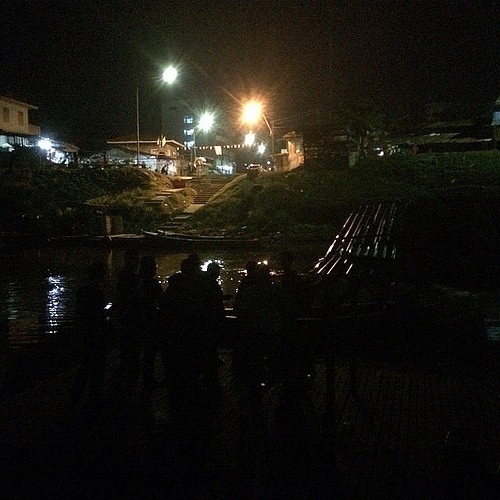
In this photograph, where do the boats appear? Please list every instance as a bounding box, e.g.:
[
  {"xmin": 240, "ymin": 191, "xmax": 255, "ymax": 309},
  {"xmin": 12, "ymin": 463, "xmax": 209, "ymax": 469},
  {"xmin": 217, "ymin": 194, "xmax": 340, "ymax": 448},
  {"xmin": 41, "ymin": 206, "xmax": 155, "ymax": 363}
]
[{"xmin": 155, "ymin": 229, "xmax": 260, "ymax": 251}]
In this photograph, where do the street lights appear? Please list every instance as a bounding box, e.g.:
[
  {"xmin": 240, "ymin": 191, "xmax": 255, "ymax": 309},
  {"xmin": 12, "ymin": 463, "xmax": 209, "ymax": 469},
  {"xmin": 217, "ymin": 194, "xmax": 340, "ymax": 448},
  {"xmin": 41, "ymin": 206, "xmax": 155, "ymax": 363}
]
[
  {"xmin": 246, "ymin": 100, "xmax": 276, "ymax": 172},
  {"xmin": 189, "ymin": 111, "xmax": 212, "ymax": 175},
  {"xmin": 136, "ymin": 66, "xmax": 178, "ymax": 169}
]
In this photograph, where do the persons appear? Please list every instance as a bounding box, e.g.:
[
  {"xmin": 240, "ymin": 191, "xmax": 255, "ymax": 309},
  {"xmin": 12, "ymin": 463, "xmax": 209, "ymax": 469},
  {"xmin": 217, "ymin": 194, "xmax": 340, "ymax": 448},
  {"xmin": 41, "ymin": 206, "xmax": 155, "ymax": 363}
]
[
  {"xmin": 67, "ymin": 261, "xmax": 109, "ymax": 417},
  {"xmin": 116, "ymin": 248, "xmax": 140, "ymax": 375},
  {"xmin": 159, "ymin": 253, "xmax": 227, "ymax": 400},
  {"xmin": 121, "ymin": 255, "xmax": 165, "ymax": 390},
  {"xmin": 237, "ymin": 250, "xmax": 365, "ymax": 409},
  {"xmin": 140, "ymin": 161, "xmax": 168, "ymax": 176}
]
